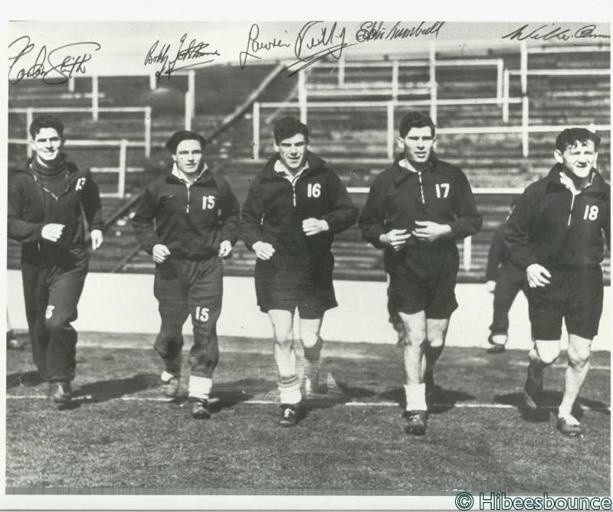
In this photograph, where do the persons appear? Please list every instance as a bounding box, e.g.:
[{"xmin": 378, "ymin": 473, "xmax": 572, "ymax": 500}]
[
  {"xmin": 357, "ymin": 111, "xmax": 483, "ymax": 434},
  {"xmin": 487, "ymin": 200, "xmax": 529, "ymax": 345},
  {"xmin": 503, "ymin": 128, "xmax": 610, "ymax": 437},
  {"xmin": 132, "ymin": 131, "xmax": 240, "ymax": 418},
  {"xmin": 8, "ymin": 120, "xmax": 104, "ymax": 402},
  {"xmin": 238, "ymin": 115, "xmax": 360, "ymax": 427}
]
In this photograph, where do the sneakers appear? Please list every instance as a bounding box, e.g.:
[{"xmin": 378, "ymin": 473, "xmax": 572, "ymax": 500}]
[
  {"xmin": 39, "ymin": 379, "xmax": 71, "ymax": 402},
  {"xmin": 488, "ymin": 331, "xmax": 507, "ymax": 347},
  {"xmin": 158, "ymin": 367, "xmax": 180, "ymax": 396},
  {"xmin": 300, "ymin": 365, "xmax": 319, "ymax": 394},
  {"xmin": 548, "ymin": 406, "xmax": 583, "ymax": 437},
  {"xmin": 7, "ymin": 337, "xmax": 24, "ymax": 350},
  {"xmin": 524, "ymin": 364, "xmax": 544, "ymax": 409},
  {"xmin": 278, "ymin": 401, "xmax": 303, "ymax": 425},
  {"xmin": 191, "ymin": 395, "xmax": 210, "ymax": 418},
  {"xmin": 403, "ymin": 409, "xmax": 427, "ymax": 435}
]
